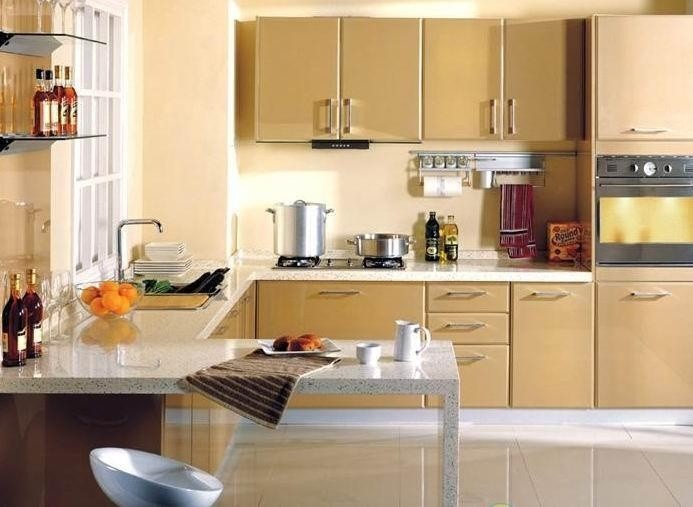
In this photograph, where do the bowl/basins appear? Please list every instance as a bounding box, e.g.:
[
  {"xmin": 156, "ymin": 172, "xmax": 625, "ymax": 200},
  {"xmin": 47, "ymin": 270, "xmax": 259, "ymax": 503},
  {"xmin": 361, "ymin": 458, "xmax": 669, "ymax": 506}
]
[
  {"xmin": 75, "ymin": 281, "xmax": 146, "ymax": 320},
  {"xmin": 80, "ymin": 320, "xmax": 142, "ymax": 349}
]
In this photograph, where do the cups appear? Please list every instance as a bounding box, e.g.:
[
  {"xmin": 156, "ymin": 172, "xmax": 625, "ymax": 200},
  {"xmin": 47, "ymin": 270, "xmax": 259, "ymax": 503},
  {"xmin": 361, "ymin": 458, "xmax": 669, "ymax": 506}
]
[
  {"xmin": 357, "ymin": 342, "xmax": 381, "ymax": 364},
  {"xmin": 392, "ymin": 320, "xmax": 431, "ymax": 362},
  {"xmin": 41, "ymin": 274, "xmax": 62, "ymax": 345},
  {"xmin": 37, "ymin": 277, "xmax": 51, "ymax": 349},
  {"xmin": 50, "ymin": 269, "xmax": 73, "ymax": 340}
]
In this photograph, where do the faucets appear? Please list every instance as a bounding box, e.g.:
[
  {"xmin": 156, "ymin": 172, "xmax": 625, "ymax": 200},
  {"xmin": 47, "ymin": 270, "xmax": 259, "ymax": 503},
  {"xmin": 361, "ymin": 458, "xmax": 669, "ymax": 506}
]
[{"xmin": 114, "ymin": 219, "xmax": 163, "ymax": 281}]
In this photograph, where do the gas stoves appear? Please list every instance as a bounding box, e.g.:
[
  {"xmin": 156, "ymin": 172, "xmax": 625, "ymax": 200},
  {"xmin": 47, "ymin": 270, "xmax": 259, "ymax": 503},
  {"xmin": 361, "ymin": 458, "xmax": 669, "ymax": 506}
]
[{"xmin": 271, "ymin": 255, "xmax": 407, "ymax": 271}]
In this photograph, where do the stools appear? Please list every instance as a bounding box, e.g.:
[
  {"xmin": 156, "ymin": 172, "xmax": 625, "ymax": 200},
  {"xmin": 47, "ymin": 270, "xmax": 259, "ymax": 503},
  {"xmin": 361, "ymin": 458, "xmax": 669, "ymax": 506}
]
[{"xmin": 90, "ymin": 447, "xmax": 223, "ymax": 506}]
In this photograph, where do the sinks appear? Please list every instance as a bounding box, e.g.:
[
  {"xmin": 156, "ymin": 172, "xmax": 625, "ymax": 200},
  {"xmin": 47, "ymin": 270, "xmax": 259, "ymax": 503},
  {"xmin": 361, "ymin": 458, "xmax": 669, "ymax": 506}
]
[{"xmin": 144, "ymin": 282, "xmax": 221, "ymax": 309}]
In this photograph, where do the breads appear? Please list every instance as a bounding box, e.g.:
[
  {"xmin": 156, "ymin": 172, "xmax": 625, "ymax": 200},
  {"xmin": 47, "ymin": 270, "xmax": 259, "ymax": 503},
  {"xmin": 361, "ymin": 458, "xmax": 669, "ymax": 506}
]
[
  {"xmin": 273, "ymin": 334, "xmax": 299, "ymax": 352},
  {"xmin": 301, "ymin": 334, "xmax": 321, "ymax": 348},
  {"xmin": 288, "ymin": 337, "xmax": 314, "ymax": 353}
]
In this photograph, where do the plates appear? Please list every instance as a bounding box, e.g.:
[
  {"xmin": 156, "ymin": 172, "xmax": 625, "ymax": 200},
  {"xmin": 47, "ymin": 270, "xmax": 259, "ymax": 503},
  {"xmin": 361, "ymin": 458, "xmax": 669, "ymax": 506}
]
[
  {"xmin": 144, "ymin": 242, "xmax": 186, "ymax": 261},
  {"xmin": 256, "ymin": 337, "xmax": 342, "ymax": 354},
  {"xmin": 134, "ymin": 254, "xmax": 193, "ymax": 278}
]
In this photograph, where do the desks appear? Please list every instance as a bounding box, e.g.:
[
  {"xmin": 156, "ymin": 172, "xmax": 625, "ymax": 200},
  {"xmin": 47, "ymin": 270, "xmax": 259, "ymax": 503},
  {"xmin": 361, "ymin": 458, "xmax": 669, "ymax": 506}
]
[{"xmin": 1, "ymin": 339, "xmax": 461, "ymax": 507}]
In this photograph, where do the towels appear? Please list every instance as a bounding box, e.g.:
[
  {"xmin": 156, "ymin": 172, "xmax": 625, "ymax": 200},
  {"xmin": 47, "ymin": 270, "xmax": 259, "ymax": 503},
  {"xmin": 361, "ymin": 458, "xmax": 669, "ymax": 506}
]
[
  {"xmin": 498, "ymin": 182, "xmax": 539, "ymax": 259},
  {"xmin": 183, "ymin": 345, "xmax": 342, "ymax": 431}
]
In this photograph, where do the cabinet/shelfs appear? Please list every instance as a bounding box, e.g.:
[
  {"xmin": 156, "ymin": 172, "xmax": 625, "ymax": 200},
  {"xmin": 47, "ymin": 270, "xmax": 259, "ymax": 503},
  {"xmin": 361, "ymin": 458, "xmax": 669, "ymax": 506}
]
[
  {"xmin": 0, "ymin": 132, "xmax": 107, "ymax": 153},
  {"xmin": 426, "ymin": 284, "xmax": 509, "ymax": 312},
  {"xmin": 512, "ymin": 284, "xmax": 596, "ymax": 408},
  {"xmin": 256, "ymin": 18, "xmax": 422, "ymax": 142},
  {"xmin": 591, "ymin": 14, "xmax": 693, "ymax": 142},
  {"xmin": 426, "ymin": 344, "xmax": 510, "ymax": 406},
  {"xmin": 594, "ymin": 279, "xmax": 692, "ymax": 408},
  {"xmin": 258, "ymin": 281, "xmax": 424, "ymax": 408},
  {"xmin": 426, "ymin": 313, "xmax": 510, "ymax": 343},
  {"xmin": 228, "ymin": 297, "xmax": 243, "ymax": 441},
  {"xmin": 421, "ymin": 20, "xmax": 585, "ymax": 141},
  {"xmin": 0, "ymin": 27, "xmax": 106, "ymax": 55}
]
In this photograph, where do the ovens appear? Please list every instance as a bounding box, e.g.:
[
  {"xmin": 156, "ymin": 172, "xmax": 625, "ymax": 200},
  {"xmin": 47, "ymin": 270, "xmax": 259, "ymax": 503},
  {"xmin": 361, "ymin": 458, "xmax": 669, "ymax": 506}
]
[{"xmin": 595, "ymin": 155, "xmax": 693, "ymax": 267}]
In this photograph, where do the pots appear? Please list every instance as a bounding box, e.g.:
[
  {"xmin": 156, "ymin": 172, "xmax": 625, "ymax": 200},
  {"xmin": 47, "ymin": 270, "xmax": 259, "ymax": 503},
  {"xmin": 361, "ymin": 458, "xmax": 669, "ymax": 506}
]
[
  {"xmin": 347, "ymin": 234, "xmax": 417, "ymax": 257},
  {"xmin": 266, "ymin": 199, "xmax": 334, "ymax": 257}
]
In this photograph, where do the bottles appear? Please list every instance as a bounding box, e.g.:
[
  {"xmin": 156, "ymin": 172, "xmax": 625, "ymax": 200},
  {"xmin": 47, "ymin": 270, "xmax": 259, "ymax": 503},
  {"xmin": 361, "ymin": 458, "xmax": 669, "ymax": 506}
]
[
  {"xmin": 53, "ymin": 65, "xmax": 69, "ymax": 136},
  {"xmin": 1, "ymin": 274, "xmax": 28, "ymax": 368},
  {"xmin": 437, "ymin": 215, "xmax": 446, "ymax": 262},
  {"xmin": 44, "ymin": 69, "xmax": 58, "ymax": 136},
  {"xmin": 22, "ymin": 268, "xmax": 43, "ymax": 359},
  {"xmin": 423, "ymin": 211, "xmax": 440, "ymax": 261},
  {"xmin": 31, "ymin": 69, "xmax": 50, "ymax": 137},
  {"xmin": 64, "ymin": 66, "xmax": 78, "ymax": 137},
  {"xmin": 444, "ymin": 216, "xmax": 458, "ymax": 261}
]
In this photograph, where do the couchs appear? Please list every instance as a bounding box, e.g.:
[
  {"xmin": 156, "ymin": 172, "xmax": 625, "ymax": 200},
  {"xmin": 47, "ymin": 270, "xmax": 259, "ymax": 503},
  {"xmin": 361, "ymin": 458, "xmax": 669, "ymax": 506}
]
[
  {"xmin": 243, "ymin": 280, "xmax": 257, "ymax": 340},
  {"xmin": 212, "ymin": 316, "xmax": 228, "ymax": 471},
  {"xmin": 191, "ymin": 392, "xmax": 212, "ymax": 472},
  {"xmin": 166, "ymin": 394, "xmax": 190, "ymax": 466}
]
